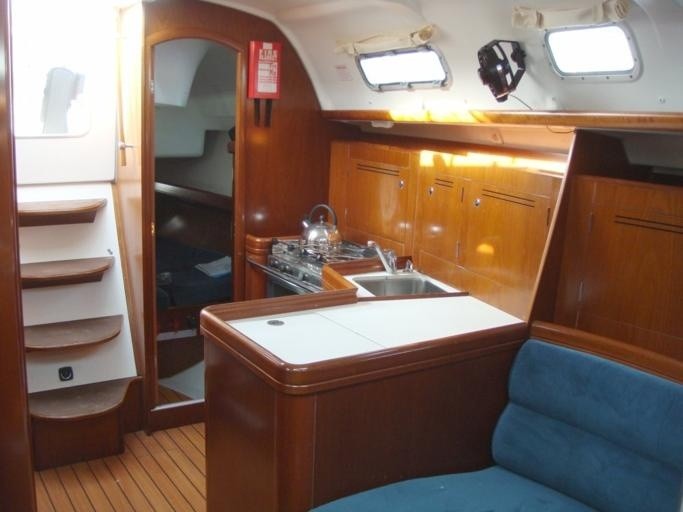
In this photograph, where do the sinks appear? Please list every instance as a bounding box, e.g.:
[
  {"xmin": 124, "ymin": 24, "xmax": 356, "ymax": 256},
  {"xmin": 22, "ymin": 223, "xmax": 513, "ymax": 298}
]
[{"xmin": 350, "ymin": 272, "xmax": 453, "ymax": 297}]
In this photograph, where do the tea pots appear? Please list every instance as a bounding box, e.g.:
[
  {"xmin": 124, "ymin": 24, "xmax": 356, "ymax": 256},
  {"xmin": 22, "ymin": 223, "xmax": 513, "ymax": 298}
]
[{"xmin": 302, "ymin": 203, "xmax": 344, "ymax": 248}]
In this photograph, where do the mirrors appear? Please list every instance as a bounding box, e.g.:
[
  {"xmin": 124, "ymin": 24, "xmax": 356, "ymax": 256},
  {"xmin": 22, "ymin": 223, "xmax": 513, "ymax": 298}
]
[{"xmin": 147, "ymin": 26, "xmax": 248, "ymax": 433}]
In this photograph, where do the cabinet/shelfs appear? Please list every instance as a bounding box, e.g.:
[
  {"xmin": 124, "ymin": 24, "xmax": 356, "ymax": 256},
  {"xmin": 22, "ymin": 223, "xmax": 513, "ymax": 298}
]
[
  {"xmin": 408, "ymin": 173, "xmax": 552, "ymax": 292},
  {"xmin": 343, "ymin": 158, "xmax": 415, "ymax": 245}
]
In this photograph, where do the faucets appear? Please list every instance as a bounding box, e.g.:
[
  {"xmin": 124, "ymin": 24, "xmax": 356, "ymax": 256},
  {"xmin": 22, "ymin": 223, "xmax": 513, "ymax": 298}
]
[{"xmin": 365, "ymin": 240, "xmax": 400, "ymax": 272}]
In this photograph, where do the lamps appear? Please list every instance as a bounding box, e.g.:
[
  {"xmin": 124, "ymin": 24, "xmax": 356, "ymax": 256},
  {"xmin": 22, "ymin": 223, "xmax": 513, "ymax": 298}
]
[{"xmin": 477, "ymin": 39, "xmax": 526, "ymax": 102}]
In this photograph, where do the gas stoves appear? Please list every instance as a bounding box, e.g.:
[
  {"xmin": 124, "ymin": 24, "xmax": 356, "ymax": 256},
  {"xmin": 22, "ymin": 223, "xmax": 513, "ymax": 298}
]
[{"xmin": 275, "ymin": 238, "xmax": 374, "ymax": 265}]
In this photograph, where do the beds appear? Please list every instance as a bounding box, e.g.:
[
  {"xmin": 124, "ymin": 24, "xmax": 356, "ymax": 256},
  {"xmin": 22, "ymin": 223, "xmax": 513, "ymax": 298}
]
[{"xmin": 306, "ymin": 320, "xmax": 682, "ymax": 512}]
